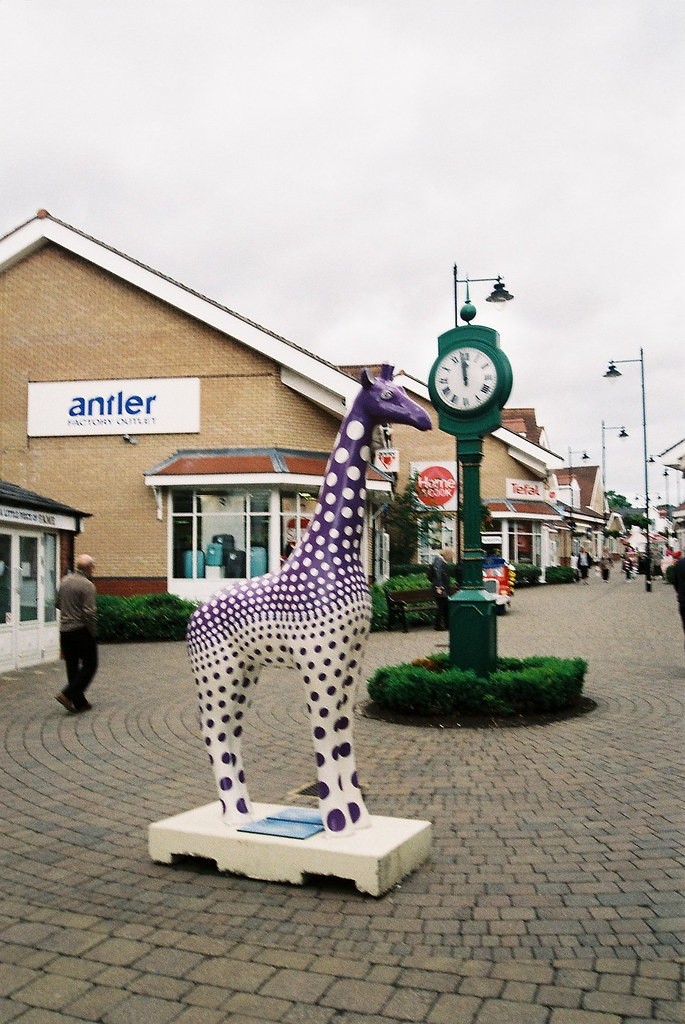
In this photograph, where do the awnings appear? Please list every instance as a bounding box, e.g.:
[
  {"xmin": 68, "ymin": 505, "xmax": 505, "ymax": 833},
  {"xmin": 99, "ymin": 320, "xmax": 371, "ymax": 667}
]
[
  {"xmin": 481, "ymin": 498, "xmax": 562, "ymax": 521},
  {"xmin": 141, "ymin": 447, "xmax": 394, "ymax": 522}
]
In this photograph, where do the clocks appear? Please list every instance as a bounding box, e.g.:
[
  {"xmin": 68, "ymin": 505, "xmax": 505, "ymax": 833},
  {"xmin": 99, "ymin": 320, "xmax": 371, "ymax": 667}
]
[{"xmin": 427, "ymin": 341, "xmax": 503, "ymax": 417}]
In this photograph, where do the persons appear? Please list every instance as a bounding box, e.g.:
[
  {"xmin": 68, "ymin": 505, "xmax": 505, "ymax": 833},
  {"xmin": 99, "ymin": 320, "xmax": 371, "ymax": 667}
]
[
  {"xmin": 53, "ymin": 554, "xmax": 98, "ymax": 714},
  {"xmin": 670, "ymin": 558, "xmax": 685, "ymax": 632},
  {"xmin": 599, "ymin": 549, "xmax": 610, "ymax": 583},
  {"xmin": 427, "ymin": 549, "xmax": 454, "ymax": 631},
  {"xmin": 577, "ymin": 547, "xmax": 593, "ymax": 579}
]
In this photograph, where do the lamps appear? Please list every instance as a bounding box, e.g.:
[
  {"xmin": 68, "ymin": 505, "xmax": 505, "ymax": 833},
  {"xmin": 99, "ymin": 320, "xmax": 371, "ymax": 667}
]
[{"xmin": 123, "ymin": 435, "xmax": 131, "ymax": 441}]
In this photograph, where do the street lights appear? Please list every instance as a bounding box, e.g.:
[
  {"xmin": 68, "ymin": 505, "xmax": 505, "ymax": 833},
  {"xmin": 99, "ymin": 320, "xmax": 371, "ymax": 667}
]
[
  {"xmin": 569, "ymin": 446, "xmax": 591, "ymax": 561},
  {"xmin": 602, "ymin": 347, "xmax": 652, "ymax": 594},
  {"xmin": 451, "ymin": 261, "xmax": 515, "ymax": 582},
  {"xmin": 601, "ymin": 419, "xmax": 629, "ymax": 582}
]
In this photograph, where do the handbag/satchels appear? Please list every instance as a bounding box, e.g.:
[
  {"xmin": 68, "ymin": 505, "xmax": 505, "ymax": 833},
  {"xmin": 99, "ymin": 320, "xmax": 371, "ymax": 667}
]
[{"xmin": 428, "ymin": 567, "xmax": 448, "ymax": 585}]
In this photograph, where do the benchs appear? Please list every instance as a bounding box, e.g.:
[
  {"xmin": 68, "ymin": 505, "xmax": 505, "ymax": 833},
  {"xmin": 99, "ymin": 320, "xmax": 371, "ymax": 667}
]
[{"xmin": 384, "ymin": 588, "xmax": 455, "ymax": 633}]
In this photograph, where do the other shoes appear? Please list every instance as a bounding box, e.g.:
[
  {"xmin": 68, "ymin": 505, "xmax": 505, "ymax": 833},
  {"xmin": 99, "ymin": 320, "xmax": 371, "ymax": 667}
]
[
  {"xmin": 56, "ymin": 693, "xmax": 75, "ymax": 713},
  {"xmin": 75, "ymin": 703, "xmax": 92, "ymax": 713},
  {"xmin": 434, "ymin": 624, "xmax": 450, "ymax": 631}
]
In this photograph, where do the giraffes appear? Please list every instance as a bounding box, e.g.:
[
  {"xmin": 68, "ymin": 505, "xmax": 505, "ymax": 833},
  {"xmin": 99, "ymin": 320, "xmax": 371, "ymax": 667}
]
[{"xmin": 187, "ymin": 368, "xmax": 432, "ymax": 836}]
